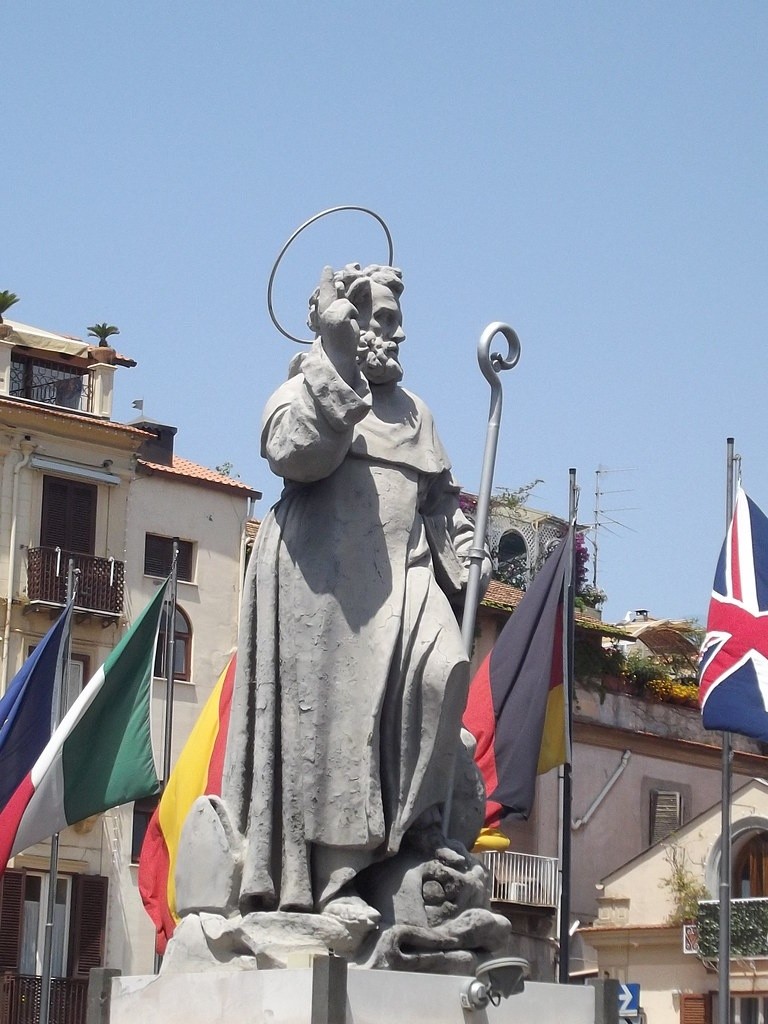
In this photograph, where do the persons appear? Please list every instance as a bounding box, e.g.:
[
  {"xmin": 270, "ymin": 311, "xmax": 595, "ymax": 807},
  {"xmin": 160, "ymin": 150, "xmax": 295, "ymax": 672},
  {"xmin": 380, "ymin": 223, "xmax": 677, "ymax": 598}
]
[{"xmin": 220, "ymin": 263, "xmax": 494, "ymax": 924}]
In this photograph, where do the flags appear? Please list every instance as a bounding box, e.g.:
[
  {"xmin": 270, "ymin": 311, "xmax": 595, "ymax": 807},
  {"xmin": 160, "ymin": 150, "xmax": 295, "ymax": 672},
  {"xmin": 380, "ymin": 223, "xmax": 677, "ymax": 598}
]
[
  {"xmin": 461, "ymin": 521, "xmax": 573, "ymax": 826},
  {"xmin": 698, "ymin": 476, "xmax": 768, "ymax": 742},
  {"xmin": 137, "ymin": 648, "xmax": 239, "ymax": 957},
  {"xmin": 0, "ymin": 573, "xmax": 172, "ymax": 880}
]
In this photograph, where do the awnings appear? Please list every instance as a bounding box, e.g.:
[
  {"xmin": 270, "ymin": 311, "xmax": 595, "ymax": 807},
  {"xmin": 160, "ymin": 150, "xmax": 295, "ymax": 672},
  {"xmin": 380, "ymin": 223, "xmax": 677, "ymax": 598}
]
[{"xmin": 617, "ymin": 618, "xmax": 701, "ymax": 675}]
